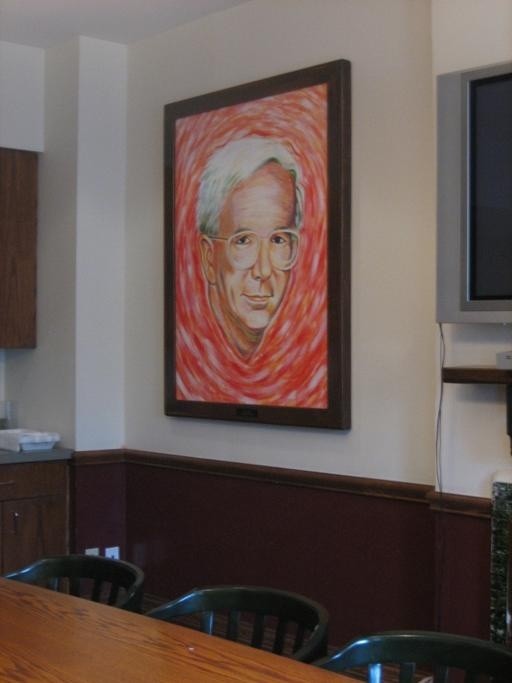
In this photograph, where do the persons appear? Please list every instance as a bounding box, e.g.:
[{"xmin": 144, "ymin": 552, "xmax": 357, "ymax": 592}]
[{"xmin": 194, "ymin": 134, "xmax": 306, "ymax": 363}]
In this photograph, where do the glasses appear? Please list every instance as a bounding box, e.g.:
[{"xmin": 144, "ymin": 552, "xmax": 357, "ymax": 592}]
[{"xmin": 207, "ymin": 230, "xmax": 300, "ymax": 271}]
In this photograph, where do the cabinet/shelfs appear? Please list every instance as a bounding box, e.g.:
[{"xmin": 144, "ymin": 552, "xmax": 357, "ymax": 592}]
[{"xmin": 0, "ymin": 459, "xmax": 70, "ymax": 593}]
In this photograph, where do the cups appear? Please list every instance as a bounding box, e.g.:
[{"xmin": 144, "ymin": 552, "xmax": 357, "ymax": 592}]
[{"xmin": 1, "ymin": 400, "xmax": 17, "ymax": 429}]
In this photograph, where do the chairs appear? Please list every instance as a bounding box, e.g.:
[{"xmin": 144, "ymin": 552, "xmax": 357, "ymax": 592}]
[
  {"xmin": 148, "ymin": 578, "xmax": 329, "ymax": 663},
  {"xmin": 4, "ymin": 557, "xmax": 146, "ymax": 612},
  {"xmin": 319, "ymin": 625, "xmax": 510, "ymax": 682}
]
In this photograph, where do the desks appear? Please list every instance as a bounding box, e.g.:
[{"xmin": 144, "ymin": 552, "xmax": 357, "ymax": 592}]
[{"xmin": 4, "ymin": 577, "xmax": 363, "ymax": 683}]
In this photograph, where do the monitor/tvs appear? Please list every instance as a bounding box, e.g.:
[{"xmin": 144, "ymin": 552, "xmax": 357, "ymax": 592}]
[{"xmin": 435, "ymin": 60, "xmax": 512, "ymax": 325}]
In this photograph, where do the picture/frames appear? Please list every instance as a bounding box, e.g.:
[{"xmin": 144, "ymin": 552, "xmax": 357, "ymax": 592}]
[{"xmin": 163, "ymin": 59, "xmax": 352, "ymax": 430}]
